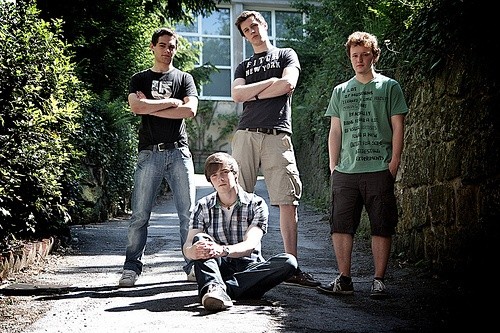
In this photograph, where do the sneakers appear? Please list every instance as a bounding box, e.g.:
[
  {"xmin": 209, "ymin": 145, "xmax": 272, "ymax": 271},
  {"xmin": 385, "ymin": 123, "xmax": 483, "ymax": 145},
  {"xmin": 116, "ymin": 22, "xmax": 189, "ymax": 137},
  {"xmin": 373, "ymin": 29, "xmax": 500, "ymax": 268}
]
[
  {"xmin": 187, "ymin": 266, "xmax": 197, "ymax": 281},
  {"xmin": 370, "ymin": 277, "xmax": 388, "ymax": 295},
  {"xmin": 119, "ymin": 269, "xmax": 137, "ymax": 286},
  {"xmin": 317, "ymin": 272, "xmax": 354, "ymax": 294}
]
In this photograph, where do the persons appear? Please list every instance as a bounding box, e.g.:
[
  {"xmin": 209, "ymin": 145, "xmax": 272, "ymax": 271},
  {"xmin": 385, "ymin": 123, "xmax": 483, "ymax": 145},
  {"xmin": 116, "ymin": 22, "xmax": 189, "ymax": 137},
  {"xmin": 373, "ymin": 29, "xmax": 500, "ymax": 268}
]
[
  {"xmin": 230, "ymin": 10, "xmax": 322, "ymax": 289},
  {"xmin": 316, "ymin": 31, "xmax": 408, "ymax": 298},
  {"xmin": 118, "ymin": 29, "xmax": 199, "ymax": 287},
  {"xmin": 183, "ymin": 152, "xmax": 299, "ymax": 311}
]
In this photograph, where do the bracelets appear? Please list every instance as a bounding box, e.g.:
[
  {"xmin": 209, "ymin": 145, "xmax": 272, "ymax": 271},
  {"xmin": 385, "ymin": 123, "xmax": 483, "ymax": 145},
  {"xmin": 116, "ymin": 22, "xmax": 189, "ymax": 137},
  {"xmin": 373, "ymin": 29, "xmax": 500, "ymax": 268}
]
[{"xmin": 255, "ymin": 95, "xmax": 259, "ymax": 100}]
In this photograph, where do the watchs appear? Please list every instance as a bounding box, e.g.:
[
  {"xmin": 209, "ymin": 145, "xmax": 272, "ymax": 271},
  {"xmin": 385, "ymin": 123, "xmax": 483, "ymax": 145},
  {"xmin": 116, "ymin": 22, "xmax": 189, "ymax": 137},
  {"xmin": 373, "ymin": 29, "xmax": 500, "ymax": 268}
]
[{"xmin": 222, "ymin": 246, "xmax": 230, "ymax": 257}]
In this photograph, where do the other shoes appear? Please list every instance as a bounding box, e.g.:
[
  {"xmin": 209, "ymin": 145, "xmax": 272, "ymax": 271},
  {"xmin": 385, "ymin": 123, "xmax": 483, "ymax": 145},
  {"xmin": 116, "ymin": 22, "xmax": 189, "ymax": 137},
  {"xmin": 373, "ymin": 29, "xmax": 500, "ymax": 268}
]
[
  {"xmin": 202, "ymin": 284, "xmax": 233, "ymax": 310},
  {"xmin": 284, "ymin": 272, "xmax": 321, "ymax": 288}
]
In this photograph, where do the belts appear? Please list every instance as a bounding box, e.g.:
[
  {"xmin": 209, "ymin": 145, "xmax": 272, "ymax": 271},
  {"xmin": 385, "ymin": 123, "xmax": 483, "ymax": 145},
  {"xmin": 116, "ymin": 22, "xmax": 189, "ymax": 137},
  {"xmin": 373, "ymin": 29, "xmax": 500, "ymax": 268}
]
[
  {"xmin": 241, "ymin": 128, "xmax": 281, "ymax": 134},
  {"xmin": 144, "ymin": 141, "xmax": 182, "ymax": 152}
]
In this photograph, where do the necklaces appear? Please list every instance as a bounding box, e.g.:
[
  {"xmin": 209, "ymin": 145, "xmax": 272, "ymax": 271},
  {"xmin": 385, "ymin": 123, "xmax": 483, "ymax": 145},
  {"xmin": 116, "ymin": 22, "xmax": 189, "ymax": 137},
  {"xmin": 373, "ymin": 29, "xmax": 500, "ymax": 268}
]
[{"xmin": 220, "ymin": 200, "xmax": 237, "ymax": 210}]
